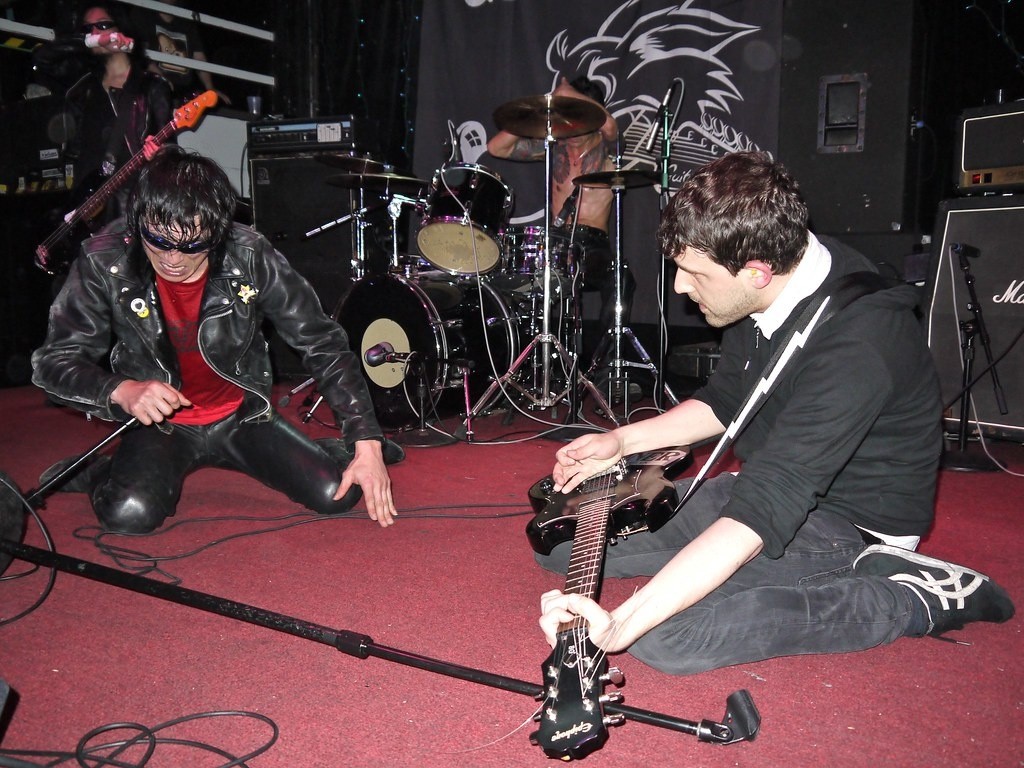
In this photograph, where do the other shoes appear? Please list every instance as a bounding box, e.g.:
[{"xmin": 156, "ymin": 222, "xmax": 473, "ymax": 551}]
[
  {"xmin": 484, "ymin": 380, "xmax": 531, "ymax": 416},
  {"xmin": 44, "ymin": 399, "xmax": 63, "ymax": 407},
  {"xmin": 313, "ymin": 437, "xmax": 406, "ymax": 465},
  {"xmin": 39, "ymin": 454, "xmax": 109, "ymax": 494},
  {"xmin": 594, "ymin": 382, "xmax": 642, "ymax": 412}
]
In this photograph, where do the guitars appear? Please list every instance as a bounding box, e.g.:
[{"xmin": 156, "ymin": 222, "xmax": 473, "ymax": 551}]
[
  {"xmin": 525, "ymin": 444, "xmax": 694, "ymax": 760},
  {"xmin": 31, "ymin": 87, "xmax": 219, "ymax": 276}
]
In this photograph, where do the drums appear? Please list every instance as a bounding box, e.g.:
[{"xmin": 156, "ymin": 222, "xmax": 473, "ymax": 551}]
[
  {"xmin": 497, "ymin": 226, "xmax": 585, "ymax": 300},
  {"xmin": 331, "ymin": 266, "xmax": 522, "ymax": 432},
  {"xmin": 415, "ymin": 164, "xmax": 514, "ymax": 275}
]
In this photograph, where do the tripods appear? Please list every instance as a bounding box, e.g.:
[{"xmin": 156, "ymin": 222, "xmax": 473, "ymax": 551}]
[{"xmin": 452, "ymin": 103, "xmax": 680, "ymax": 441}]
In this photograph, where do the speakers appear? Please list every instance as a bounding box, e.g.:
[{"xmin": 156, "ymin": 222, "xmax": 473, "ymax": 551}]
[
  {"xmin": 248, "ymin": 151, "xmax": 357, "ymax": 280},
  {"xmin": 778, "ymin": 1, "xmax": 929, "ymax": 295},
  {"xmin": 919, "ymin": 192, "xmax": 1023, "ymax": 443},
  {"xmin": 956, "ymin": 100, "xmax": 1024, "ymax": 196}
]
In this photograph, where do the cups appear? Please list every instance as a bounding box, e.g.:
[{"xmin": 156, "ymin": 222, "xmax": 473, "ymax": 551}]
[{"xmin": 247, "ymin": 96, "xmax": 261, "ymax": 115}]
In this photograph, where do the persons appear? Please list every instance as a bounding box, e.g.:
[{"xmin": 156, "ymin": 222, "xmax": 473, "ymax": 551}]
[
  {"xmin": 537, "ymin": 153, "xmax": 1016, "ymax": 675},
  {"xmin": 65, "ymin": 3, "xmax": 176, "ymax": 235},
  {"xmin": 487, "ymin": 77, "xmax": 641, "ymax": 407},
  {"xmin": 141, "ymin": 0, "xmax": 231, "ymax": 105},
  {"xmin": 32, "ymin": 141, "xmax": 404, "ymax": 534}
]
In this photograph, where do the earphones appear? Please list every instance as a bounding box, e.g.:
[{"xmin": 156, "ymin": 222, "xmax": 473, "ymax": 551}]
[{"xmin": 750, "ymin": 269, "xmax": 762, "ymax": 277}]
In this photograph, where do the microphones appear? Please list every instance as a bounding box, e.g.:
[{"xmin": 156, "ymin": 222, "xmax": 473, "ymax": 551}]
[
  {"xmin": 554, "ymin": 185, "xmax": 579, "ymax": 227},
  {"xmin": 305, "ymin": 205, "xmax": 374, "ymax": 238},
  {"xmin": 366, "ymin": 342, "xmax": 394, "ymax": 367},
  {"xmin": 452, "ymin": 126, "xmax": 461, "ymax": 162},
  {"xmin": 949, "ymin": 242, "xmax": 981, "ymax": 258},
  {"xmin": 647, "ymin": 80, "xmax": 675, "ymax": 151}
]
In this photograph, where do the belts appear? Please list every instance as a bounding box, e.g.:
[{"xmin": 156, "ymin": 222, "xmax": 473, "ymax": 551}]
[
  {"xmin": 850, "ymin": 523, "xmax": 881, "ymax": 545},
  {"xmin": 550, "ymin": 224, "xmax": 608, "ymax": 239}
]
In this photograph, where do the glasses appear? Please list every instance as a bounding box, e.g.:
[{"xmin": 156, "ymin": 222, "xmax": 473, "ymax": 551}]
[
  {"xmin": 139, "ymin": 214, "xmax": 212, "ymax": 254},
  {"xmin": 80, "ymin": 21, "xmax": 116, "ymax": 34}
]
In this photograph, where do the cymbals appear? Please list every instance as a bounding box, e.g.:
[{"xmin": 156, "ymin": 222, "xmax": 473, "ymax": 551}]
[
  {"xmin": 493, "ymin": 95, "xmax": 607, "ymax": 138},
  {"xmin": 314, "ymin": 152, "xmax": 386, "ymax": 170},
  {"xmin": 323, "ymin": 173, "xmax": 431, "ymax": 193},
  {"xmin": 572, "ymin": 168, "xmax": 663, "ymax": 187}
]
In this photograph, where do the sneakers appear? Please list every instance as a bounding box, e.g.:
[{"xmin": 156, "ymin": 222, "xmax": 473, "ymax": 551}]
[{"xmin": 852, "ymin": 544, "xmax": 1016, "ymax": 637}]
